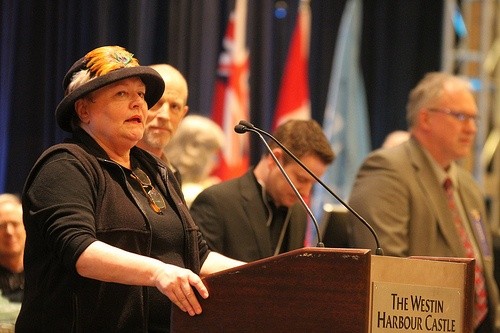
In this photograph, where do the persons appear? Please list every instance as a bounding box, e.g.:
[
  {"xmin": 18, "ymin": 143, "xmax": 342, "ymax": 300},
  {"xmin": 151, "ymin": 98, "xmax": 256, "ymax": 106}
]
[
  {"xmin": 186, "ymin": 119, "xmax": 335, "ymax": 272},
  {"xmin": 132, "ymin": 63, "xmax": 189, "ymax": 191},
  {"xmin": 349, "ymin": 72, "xmax": 500, "ymax": 333},
  {"xmin": 14, "ymin": 44, "xmax": 252, "ymax": 333},
  {"xmin": 1, "ymin": 193, "xmax": 27, "ymax": 333},
  {"xmin": 162, "ymin": 114, "xmax": 227, "ymax": 213}
]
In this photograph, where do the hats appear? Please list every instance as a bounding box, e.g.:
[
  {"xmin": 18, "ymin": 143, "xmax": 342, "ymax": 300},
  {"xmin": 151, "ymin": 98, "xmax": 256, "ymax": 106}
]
[{"xmin": 55, "ymin": 45, "xmax": 165, "ymax": 133}]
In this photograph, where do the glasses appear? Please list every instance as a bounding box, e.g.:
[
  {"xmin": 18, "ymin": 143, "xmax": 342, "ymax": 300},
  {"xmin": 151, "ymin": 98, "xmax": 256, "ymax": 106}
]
[
  {"xmin": 129, "ymin": 167, "xmax": 167, "ymax": 215},
  {"xmin": 426, "ymin": 106, "xmax": 481, "ymax": 125}
]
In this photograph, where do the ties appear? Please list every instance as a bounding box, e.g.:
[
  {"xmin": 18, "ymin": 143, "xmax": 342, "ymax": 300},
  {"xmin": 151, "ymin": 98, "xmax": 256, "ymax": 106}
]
[{"xmin": 443, "ymin": 176, "xmax": 489, "ymax": 328}]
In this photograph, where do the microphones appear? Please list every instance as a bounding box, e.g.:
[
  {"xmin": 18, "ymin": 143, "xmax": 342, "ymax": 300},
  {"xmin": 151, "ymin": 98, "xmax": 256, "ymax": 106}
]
[
  {"xmin": 234, "ymin": 125, "xmax": 325, "ymax": 247},
  {"xmin": 238, "ymin": 120, "xmax": 385, "ymax": 256}
]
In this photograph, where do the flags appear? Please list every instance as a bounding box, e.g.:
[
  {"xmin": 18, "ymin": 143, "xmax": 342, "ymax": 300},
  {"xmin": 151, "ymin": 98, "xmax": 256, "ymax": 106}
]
[
  {"xmin": 212, "ymin": 0, "xmax": 256, "ymax": 183},
  {"xmin": 274, "ymin": 0, "xmax": 312, "ymax": 139},
  {"xmin": 309, "ymin": 0, "xmax": 374, "ymax": 253}
]
